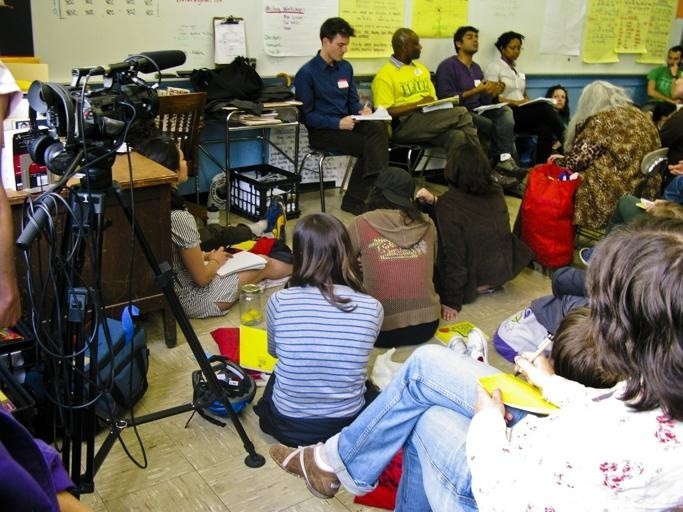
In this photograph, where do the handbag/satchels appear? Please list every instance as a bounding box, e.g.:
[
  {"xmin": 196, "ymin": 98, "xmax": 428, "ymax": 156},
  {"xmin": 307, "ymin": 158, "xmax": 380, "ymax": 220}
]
[
  {"xmin": 65, "ymin": 318, "xmax": 151, "ymax": 425},
  {"xmin": 519, "ymin": 160, "xmax": 580, "ymax": 273},
  {"xmin": 192, "ymin": 56, "xmax": 264, "ymax": 103}
]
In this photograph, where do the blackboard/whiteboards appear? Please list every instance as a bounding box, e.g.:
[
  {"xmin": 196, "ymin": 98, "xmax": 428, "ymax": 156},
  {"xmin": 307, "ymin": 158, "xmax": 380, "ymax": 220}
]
[{"xmin": 31, "ymin": 0, "xmax": 683, "ymax": 85}]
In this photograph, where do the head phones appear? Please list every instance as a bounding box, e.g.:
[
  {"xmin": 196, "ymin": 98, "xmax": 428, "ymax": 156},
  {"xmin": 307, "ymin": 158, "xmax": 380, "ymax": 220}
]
[{"xmin": 28, "ymin": 81, "xmax": 74, "ymax": 174}]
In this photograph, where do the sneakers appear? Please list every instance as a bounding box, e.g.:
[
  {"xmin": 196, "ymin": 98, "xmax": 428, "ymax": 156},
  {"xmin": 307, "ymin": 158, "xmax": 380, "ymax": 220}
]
[
  {"xmin": 269, "ymin": 442, "xmax": 341, "ymax": 500},
  {"xmin": 271, "ymin": 201, "xmax": 286, "ymax": 243},
  {"xmin": 449, "ymin": 327, "xmax": 487, "ymax": 364},
  {"xmin": 497, "ymin": 157, "xmax": 520, "ymax": 172},
  {"xmin": 341, "ymin": 197, "xmax": 367, "ymax": 214},
  {"xmin": 489, "ymin": 169, "xmax": 521, "ymax": 188}
]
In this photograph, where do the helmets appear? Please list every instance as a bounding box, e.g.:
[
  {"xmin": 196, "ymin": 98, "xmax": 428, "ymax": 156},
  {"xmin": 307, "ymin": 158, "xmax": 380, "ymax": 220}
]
[{"xmin": 192, "ymin": 361, "xmax": 256, "ymax": 419}]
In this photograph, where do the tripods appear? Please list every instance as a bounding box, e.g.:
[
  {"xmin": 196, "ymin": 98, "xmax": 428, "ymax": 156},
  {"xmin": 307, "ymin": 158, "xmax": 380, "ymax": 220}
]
[{"xmin": 39, "ymin": 182, "xmax": 265, "ymax": 501}]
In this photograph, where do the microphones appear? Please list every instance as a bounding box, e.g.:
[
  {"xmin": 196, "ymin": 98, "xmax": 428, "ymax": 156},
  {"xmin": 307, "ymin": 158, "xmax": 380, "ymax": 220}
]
[{"xmin": 106, "ymin": 50, "xmax": 186, "ymax": 73}]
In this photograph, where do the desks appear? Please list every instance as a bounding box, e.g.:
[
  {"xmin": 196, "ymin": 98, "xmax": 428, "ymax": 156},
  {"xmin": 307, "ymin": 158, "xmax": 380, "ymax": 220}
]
[
  {"xmin": 195, "ymin": 96, "xmax": 303, "ymax": 228},
  {"xmin": 7, "ymin": 118, "xmax": 178, "ymax": 351}
]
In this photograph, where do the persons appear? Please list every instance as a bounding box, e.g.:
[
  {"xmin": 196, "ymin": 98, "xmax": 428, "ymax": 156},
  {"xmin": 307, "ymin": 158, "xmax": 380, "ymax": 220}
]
[
  {"xmin": 124, "ymin": 121, "xmax": 294, "ymax": 319},
  {"xmin": 548, "ymin": 44, "xmax": 682, "ymax": 265},
  {"xmin": 1, "ymin": 54, "xmax": 94, "ymax": 511},
  {"xmin": 293, "ymin": 15, "xmax": 571, "ymax": 216},
  {"xmin": 347, "ymin": 143, "xmax": 536, "ymax": 347},
  {"xmin": 252, "ymin": 212, "xmax": 384, "ymax": 448},
  {"xmin": 268, "ymin": 203, "xmax": 682, "ymax": 512}
]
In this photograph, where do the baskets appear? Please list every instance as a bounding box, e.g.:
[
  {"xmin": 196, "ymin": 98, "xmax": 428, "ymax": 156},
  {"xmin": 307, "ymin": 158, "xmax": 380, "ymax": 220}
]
[{"xmin": 231, "ymin": 163, "xmax": 301, "ymax": 222}]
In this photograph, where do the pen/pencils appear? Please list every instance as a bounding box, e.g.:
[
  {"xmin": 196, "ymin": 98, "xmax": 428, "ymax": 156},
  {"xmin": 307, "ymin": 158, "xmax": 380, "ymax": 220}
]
[
  {"xmin": 365, "ymin": 101, "xmax": 369, "ymax": 107},
  {"xmin": 421, "ymin": 95, "xmax": 425, "ymax": 98},
  {"xmin": 515, "ymin": 333, "xmax": 557, "ymax": 377}
]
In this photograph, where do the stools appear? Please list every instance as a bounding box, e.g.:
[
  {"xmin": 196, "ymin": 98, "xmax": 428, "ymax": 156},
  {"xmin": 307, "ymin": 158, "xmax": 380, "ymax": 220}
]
[
  {"xmin": 297, "ymin": 152, "xmax": 359, "ymax": 214},
  {"xmin": 388, "ymin": 140, "xmax": 451, "ymax": 185}
]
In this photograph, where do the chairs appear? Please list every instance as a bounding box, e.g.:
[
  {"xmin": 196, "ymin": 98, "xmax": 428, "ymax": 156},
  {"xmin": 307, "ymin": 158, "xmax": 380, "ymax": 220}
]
[
  {"xmin": 572, "ymin": 146, "xmax": 670, "ymax": 258},
  {"xmin": 636, "ymin": 98, "xmax": 677, "ymax": 126},
  {"xmin": 155, "ymin": 91, "xmax": 208, "ymax": 226}
]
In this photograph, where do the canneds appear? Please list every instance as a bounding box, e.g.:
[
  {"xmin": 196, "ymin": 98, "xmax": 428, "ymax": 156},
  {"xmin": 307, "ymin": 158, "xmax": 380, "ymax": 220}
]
[{"xmin": 238, "ymin": 282, "xmax": 263, "ymax": 326}]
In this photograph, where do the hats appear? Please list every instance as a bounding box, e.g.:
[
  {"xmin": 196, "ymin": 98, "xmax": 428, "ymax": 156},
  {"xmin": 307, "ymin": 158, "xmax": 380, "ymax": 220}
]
[{"xmin": 376, "ymin": 165, "xmax": 414, "ymax": 208}]
